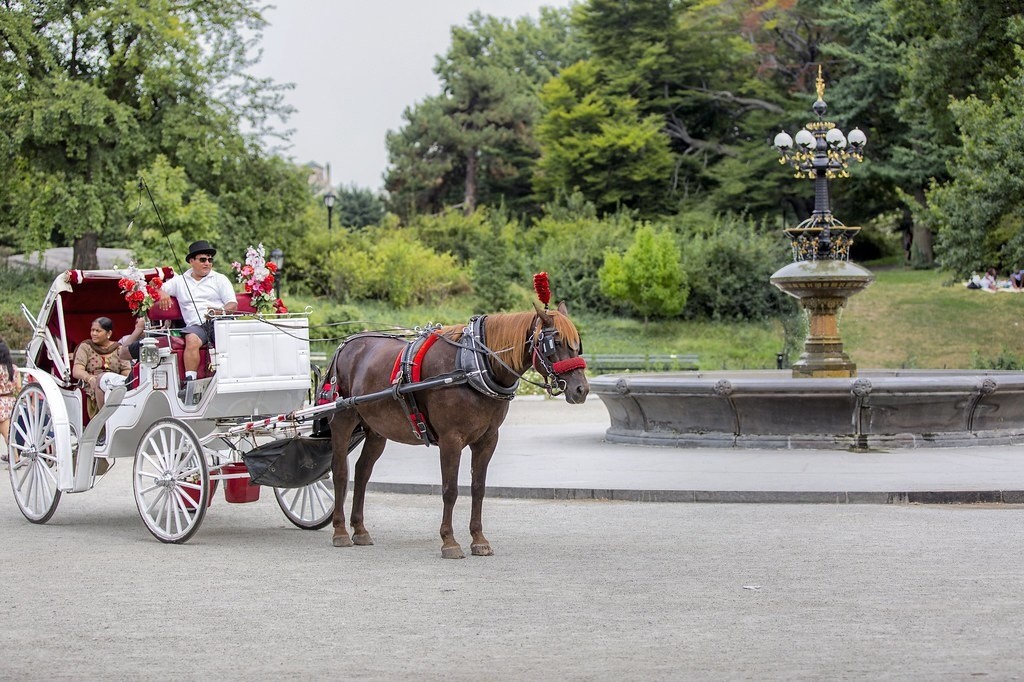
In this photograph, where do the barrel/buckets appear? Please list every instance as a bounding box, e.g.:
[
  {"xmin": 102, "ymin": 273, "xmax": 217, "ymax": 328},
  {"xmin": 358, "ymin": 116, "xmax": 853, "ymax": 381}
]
[
  {"xmin": 220, "ymin": 462, "xmax": 260, "ymax": 504},
  {"xmin": 177, "ymin": 471, "xmax": 218, "ymax": 508}
]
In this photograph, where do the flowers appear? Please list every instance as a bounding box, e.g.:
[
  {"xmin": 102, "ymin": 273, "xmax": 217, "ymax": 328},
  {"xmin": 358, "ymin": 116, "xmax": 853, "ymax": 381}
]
[
  {"xmin": 113, "ymin": 261, "xmax": 163, "ymax": 364},
  {"xmin": 232, "ymin": 242, "xmax": 288, "ymax": 319}
]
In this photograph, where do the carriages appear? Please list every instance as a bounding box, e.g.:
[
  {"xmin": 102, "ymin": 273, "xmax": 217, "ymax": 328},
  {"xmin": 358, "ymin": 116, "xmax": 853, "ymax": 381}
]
[{"xmin": 7, "ymin": 264, "xmax": 589, "ymax": 560}]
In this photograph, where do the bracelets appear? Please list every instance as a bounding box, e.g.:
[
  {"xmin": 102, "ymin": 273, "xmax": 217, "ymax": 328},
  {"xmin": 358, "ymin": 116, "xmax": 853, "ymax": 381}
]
[
  {"xmin": 221, "ymin": 307, "xmax": 227, "ymax": 315},
  {"xmin": 88, "ymin": 377, "xmax": 93, "ymax": 382}
]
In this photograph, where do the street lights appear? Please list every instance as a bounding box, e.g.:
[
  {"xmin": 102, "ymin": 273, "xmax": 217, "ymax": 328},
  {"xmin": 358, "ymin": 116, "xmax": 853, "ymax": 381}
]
[
  {"xmin": 268, "ymin": 247, "xmax": 283, "ymax": 320},
  {"xmin": 324, "ymin": 193, "xmax": 336, "ymax": 256},
  {"xmin": 771, "ymin": 63, "xmax": 871, "ymax": 375}
]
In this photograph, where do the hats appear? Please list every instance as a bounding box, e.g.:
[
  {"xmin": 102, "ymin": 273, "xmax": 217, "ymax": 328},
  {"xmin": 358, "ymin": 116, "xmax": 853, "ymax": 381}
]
[{"xmin": 185, "ymin": 240, "xmax": 216, "ymax": 264}]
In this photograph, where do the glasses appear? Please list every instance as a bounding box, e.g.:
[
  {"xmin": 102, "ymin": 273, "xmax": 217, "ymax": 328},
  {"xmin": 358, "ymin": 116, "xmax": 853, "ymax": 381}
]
[{"xmin": 194, "ymin": 257, "xmax": 214, "ymax": 263}]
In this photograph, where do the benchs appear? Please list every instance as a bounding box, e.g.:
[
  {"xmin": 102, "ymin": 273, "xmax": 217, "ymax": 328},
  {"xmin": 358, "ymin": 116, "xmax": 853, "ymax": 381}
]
[
  {"xmin": 51, "ymin": 352, "xmax": 90, "ymax": 427},
  {"xmin": 147, "ymin": 293, "xmax": 257, "ymax": 349}
]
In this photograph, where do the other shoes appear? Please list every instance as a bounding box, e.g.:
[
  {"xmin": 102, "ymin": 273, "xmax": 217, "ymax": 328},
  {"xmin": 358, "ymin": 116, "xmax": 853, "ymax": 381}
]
[
  {"xmin": 1, "ymin": 455, "xmax": 9, "ymax": 461},
  {"xmin": 178, "ymin": 375, "xmax": 198, "ymax": 398},
  {"xmin": 5, "ymin": 460, "xmax": 22, "ymax": 471}
]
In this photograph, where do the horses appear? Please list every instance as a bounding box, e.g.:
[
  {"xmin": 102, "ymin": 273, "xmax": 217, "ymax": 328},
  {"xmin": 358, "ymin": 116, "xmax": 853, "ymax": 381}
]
[{"xmin": 313, "ymin": 301, "xmax": 590, "ymax": 560}]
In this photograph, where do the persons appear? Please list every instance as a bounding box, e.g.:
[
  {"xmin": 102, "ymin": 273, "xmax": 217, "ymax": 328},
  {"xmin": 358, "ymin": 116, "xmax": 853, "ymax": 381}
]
[
  {"xmin": 980, "ymin": 264, "xmax": 1024, "ymax": 290},
  {"xmin": 0, "ymin": 343, "xmax": 53, "ymax": 470},
  {"xmin": 73, "ymin": 313, "xmax": 167, "ymax": 446},
  {"xmin": 155, "ymin": 240, "xmax": 239, "ymax": 399}
]
